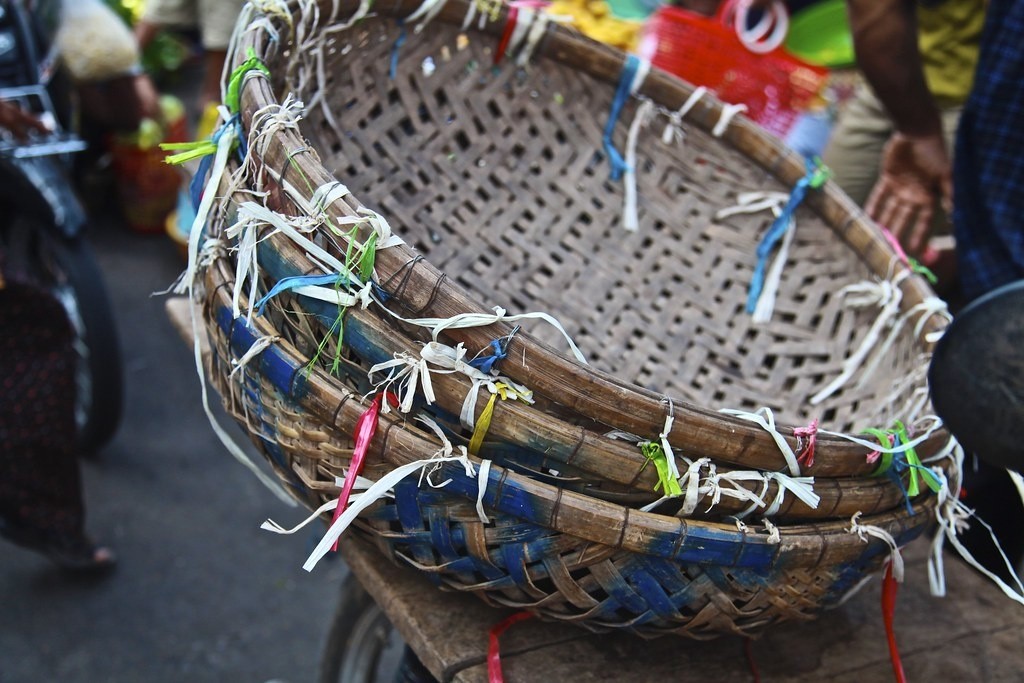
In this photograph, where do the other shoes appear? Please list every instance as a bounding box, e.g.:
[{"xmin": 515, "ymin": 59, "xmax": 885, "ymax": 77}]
[{"xmin": 0, "ymin": 520, "xmax": 116, "ymax": 576}]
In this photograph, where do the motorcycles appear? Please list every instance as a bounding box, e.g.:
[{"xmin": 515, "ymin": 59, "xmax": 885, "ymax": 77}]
[{"xmin": 1, "ymin": 0, "xmax": 126, "ymax": 456}]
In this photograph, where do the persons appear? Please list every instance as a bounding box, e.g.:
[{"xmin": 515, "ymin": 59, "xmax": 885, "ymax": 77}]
[
  {"xmin": 852, "ymin": 0, "xmax": 1023, "ymax": 583},
  {"xmin": 2, "ymin": 0, "xmax": 264, "ymax": 195},
  {"xmin": 813, "ymin": 1, "xmax": 987, "ymax": 243}
]
